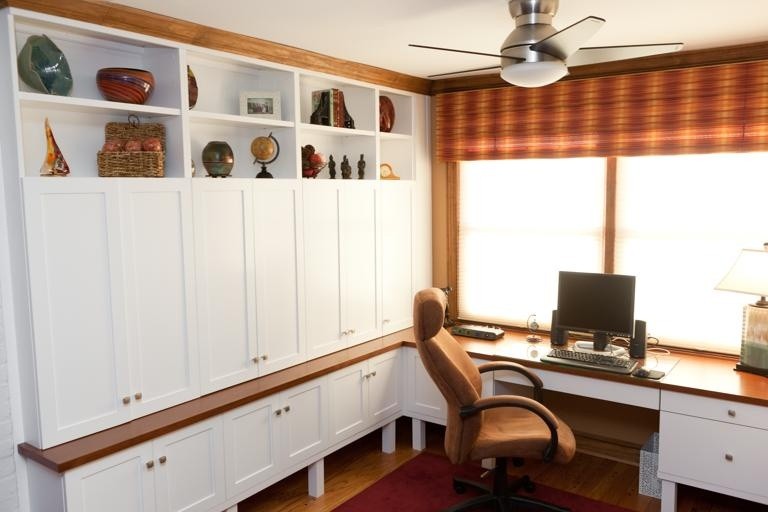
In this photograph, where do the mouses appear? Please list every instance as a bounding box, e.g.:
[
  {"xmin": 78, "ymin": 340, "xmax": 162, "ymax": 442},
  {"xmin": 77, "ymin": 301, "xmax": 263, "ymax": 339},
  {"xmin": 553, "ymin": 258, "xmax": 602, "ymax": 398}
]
[{"xmin": 637, "ymin": 366, "xmax": 650, "ymax": 376}]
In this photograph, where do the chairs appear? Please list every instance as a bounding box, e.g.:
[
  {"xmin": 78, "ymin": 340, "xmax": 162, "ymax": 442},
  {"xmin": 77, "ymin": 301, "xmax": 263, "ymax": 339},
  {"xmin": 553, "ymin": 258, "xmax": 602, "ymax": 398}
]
[{"xmin": 411, "ymin": 287, "xmax": 577, "ymax": 512}]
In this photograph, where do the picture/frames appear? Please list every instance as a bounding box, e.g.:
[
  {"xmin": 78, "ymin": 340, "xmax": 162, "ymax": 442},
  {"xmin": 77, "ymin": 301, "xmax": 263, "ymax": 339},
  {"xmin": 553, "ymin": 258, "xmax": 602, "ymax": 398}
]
[
  {"xmin": 183, "ymin": 40, "xmax": 303, "ymax": 399},
  {"xmin": 297, "ymin": 66, "xmax": 379, "ymax": 366},
  {"xmin": 376, "ymin": 81, "xmax": 432, "ymax": 340},
  {"xmin": 237, "ymin": 90, "xmax": 281, "ymax": 121}
]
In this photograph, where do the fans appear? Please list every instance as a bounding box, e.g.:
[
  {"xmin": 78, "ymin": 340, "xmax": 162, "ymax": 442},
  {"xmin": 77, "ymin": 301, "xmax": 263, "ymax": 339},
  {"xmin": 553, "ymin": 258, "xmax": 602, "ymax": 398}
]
[{"xmin": 405, "ymin": 0, "xmax": 684, "ymax": 90}]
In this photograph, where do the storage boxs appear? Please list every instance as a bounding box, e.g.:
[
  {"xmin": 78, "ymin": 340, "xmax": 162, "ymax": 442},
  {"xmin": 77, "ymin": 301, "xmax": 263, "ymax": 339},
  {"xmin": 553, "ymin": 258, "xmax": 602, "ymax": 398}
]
[{"xmin": 635, "ymin": 429, "xmax": 694, "ymax": 502}]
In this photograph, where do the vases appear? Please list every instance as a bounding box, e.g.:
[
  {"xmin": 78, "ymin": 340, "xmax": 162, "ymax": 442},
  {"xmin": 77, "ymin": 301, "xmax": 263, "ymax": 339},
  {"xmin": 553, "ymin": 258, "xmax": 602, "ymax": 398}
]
[
  {"xmin": 378, "ymin": 93, "xmax": 395, "ymax": 132},
  {"xmin": 185, "ymin": 64, "xmax": 199, "ymax": 110}
]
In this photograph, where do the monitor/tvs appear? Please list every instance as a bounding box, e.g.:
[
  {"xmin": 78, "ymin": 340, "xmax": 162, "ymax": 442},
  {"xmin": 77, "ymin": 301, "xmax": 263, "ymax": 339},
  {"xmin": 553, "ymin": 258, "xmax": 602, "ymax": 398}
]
[{"xmin": 556, "ymin": 271, "xmax": 636, "ymax": 356}]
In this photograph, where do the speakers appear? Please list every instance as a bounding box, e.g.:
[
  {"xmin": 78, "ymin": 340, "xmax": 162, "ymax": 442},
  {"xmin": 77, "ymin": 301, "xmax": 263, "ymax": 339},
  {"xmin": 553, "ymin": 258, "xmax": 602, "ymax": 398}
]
[
  {"xmin": 630, "ymin": 319, "xmax": 646, "ymax": 358},
  {"xmin": 551, "ymin": 310, "xmax": 568, "ymax": 346}
]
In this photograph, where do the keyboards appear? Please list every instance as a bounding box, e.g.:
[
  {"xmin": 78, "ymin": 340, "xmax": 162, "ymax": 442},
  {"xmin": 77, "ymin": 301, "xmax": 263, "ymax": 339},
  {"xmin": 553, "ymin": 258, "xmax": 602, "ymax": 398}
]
[{"xmin": 540, "ymin": 348, "xmax": 638, "ymax": 374}]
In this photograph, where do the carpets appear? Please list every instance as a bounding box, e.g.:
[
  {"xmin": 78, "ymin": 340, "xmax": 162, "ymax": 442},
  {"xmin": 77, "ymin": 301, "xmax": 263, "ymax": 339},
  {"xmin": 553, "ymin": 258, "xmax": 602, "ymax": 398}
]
[{"xmin": 328, "ymin": 448, "xmax": 641, "ymax": 512}]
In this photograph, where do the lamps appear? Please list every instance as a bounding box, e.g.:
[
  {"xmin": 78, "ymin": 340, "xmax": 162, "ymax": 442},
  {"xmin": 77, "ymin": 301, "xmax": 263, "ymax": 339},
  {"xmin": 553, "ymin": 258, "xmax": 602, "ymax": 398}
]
[
  {"xmin": 497, "ymin": 60, "xmax": 568, "ymax": 90},
  {"xmin": 711, "ymin": 243, "xmax": 767, "ymax": 378}
]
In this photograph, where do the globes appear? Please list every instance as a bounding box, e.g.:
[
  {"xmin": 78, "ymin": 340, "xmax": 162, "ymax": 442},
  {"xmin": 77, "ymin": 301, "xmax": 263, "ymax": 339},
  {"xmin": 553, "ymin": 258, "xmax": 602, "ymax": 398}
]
[{"xmin": 251, "ymin": 132, "xmax": 279, "ymax": 178}]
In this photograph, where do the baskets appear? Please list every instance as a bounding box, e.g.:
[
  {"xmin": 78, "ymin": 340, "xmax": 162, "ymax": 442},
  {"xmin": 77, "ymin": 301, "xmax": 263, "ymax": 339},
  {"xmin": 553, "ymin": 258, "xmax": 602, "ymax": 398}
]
[{"xmin": 95, "ymin": 120, "xmax": 170, "ymax": 179}]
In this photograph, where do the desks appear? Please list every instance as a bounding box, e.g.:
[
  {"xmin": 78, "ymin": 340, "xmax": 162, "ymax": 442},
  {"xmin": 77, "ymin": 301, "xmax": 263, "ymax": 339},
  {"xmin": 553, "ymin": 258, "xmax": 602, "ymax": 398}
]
[{"xmin": 403, "ymin": 314, "xmax": 767, "ymax": 512}]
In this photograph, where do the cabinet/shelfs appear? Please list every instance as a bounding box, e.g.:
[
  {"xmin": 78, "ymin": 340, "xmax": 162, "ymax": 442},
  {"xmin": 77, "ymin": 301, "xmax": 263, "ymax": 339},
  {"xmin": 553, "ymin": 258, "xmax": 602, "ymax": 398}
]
[
  {"xmin": 325, "ymin": 343, "xmax": 406, "ymax": 456},
  {"xmin": 16, "ymin": 415, "xmax": 226, "ymax": 512},
  {"xmin": 220, "ymin": 375, "xmax": 325, "ymax": 512},
  {"xmin": 0, "ymin": 7, "xmax": 195, "ymax": 449}
]
